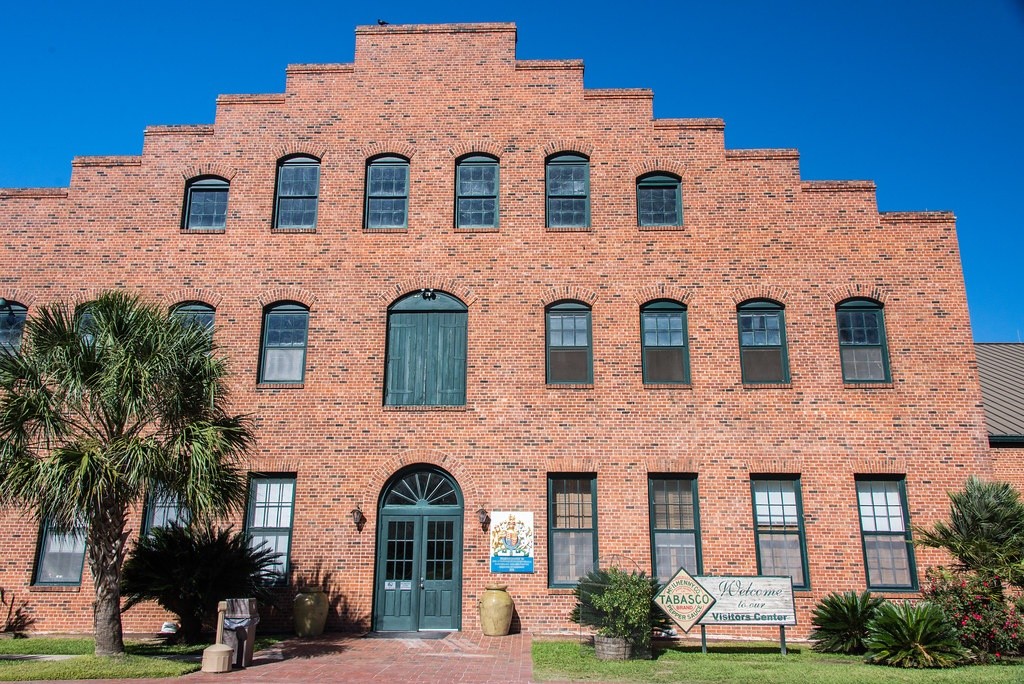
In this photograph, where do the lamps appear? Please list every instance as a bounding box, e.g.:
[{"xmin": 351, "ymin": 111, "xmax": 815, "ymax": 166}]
[
  {"xmin": 351, "ymin": 505, "xmax": 363, "ymax": 526},
  {"xmin": 476, "ymin": 508, "xmax": 488, "ymax": 529}
]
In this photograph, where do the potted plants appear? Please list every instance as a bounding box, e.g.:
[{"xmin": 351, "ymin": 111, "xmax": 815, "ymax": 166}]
[{"xmin": 590, "ymin": 566, "xmax": 652, "ymax": 660}]
